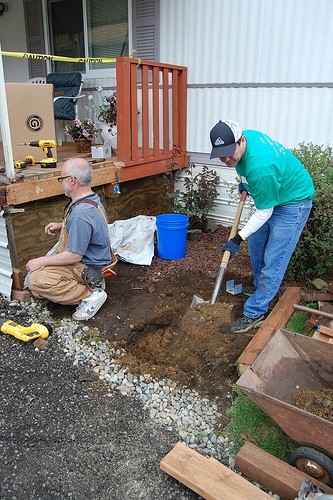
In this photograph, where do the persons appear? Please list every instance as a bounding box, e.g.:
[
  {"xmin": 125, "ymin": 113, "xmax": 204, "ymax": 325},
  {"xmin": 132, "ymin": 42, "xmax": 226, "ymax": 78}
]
[
  {"xmin": 210, "ymin": 119, "xmax": 315, "ymax": 333},
  {"xmin": 25, "ymin": 157, "xmax": 112, "ymax": 320}
]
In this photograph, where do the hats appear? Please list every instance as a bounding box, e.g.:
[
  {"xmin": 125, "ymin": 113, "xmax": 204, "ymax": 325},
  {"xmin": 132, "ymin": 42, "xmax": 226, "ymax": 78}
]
[{"xmin": 210, "ymin": 120, "xmax": 242, "ymax": 159}]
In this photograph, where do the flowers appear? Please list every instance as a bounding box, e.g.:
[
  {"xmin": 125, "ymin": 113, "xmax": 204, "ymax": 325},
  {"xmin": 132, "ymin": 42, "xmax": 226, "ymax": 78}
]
[
  {"xmin": 86, "ymin": 86, "xmax": 117, "ymax": 135},
  {"xmin": 64, "ymin": 117, "xmax": 102, "ymax": 141}
]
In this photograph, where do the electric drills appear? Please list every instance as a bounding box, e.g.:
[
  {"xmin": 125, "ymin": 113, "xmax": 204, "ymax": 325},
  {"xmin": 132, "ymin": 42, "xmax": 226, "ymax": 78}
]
[
  {"xmin": 0, "ymin": 318, "xmax": 52, "ymax": 342},
  {"xmin": 17, "ymin": 140, "xmax": 57, "ymax": 158}
]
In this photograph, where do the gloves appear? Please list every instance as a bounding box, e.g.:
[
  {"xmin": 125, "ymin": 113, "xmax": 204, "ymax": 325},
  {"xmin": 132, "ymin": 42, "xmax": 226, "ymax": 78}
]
[
  {"xmin": 239, "ymin": 182, "xmax": 250, "ymax": 195},
  {"xmin": 221, "ymin": 240, "xmax": 240, "ymax": 258}
]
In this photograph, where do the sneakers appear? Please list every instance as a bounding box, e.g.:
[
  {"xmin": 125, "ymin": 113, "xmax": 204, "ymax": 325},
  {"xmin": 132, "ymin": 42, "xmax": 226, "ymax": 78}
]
[
  {"xmin": 229, "ymin": 314, "xmax": 265, "ymax": 333},
  {"xmin": 96, "ymin": 278, "xmax": 105, "ymax": 290},
  {"xmin": 72, "ymin": 288, "xmax": 107, "ymax": 320},
  {"xmin": 243, "ymin": 285, "xmax": 256, "ymax": 296}
]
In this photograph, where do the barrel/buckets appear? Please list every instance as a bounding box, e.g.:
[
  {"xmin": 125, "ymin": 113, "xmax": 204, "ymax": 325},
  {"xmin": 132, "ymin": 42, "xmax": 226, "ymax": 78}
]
[{"xmin": 156, "ymin": 214, "xmax": 189, "ymax": 260}]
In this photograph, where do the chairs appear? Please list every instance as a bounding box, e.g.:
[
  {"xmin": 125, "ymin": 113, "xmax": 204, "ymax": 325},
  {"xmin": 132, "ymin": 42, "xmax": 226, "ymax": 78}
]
[{"xmin": 28, "ymin": 72, "xmax": 86, "ymax": 146}]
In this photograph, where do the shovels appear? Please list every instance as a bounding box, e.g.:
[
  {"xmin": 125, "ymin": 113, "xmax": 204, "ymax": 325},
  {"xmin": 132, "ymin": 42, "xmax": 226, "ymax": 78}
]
[{"xmin": 189, "ymin": 191, "xmax": 248, "ymax": 321}]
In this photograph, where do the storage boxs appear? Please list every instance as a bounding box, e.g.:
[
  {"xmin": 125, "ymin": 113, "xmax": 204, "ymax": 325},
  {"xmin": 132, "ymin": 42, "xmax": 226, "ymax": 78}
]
[{"xmin": 91, "ymin": 146, "xmax": 111, "ymax": 158}]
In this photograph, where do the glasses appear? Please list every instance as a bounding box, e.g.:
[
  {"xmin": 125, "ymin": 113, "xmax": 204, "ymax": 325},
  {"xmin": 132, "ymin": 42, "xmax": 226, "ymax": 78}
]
[{"xmin": 57, "ymin": 176, "xmax": 76, "ymax": 184}]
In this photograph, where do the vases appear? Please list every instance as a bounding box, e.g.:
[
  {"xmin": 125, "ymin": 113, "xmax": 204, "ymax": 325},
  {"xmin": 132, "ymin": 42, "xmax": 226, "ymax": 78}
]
[{"xmin": 75, "ymin": 139, "xmax": 92, "ymax": 153}]
